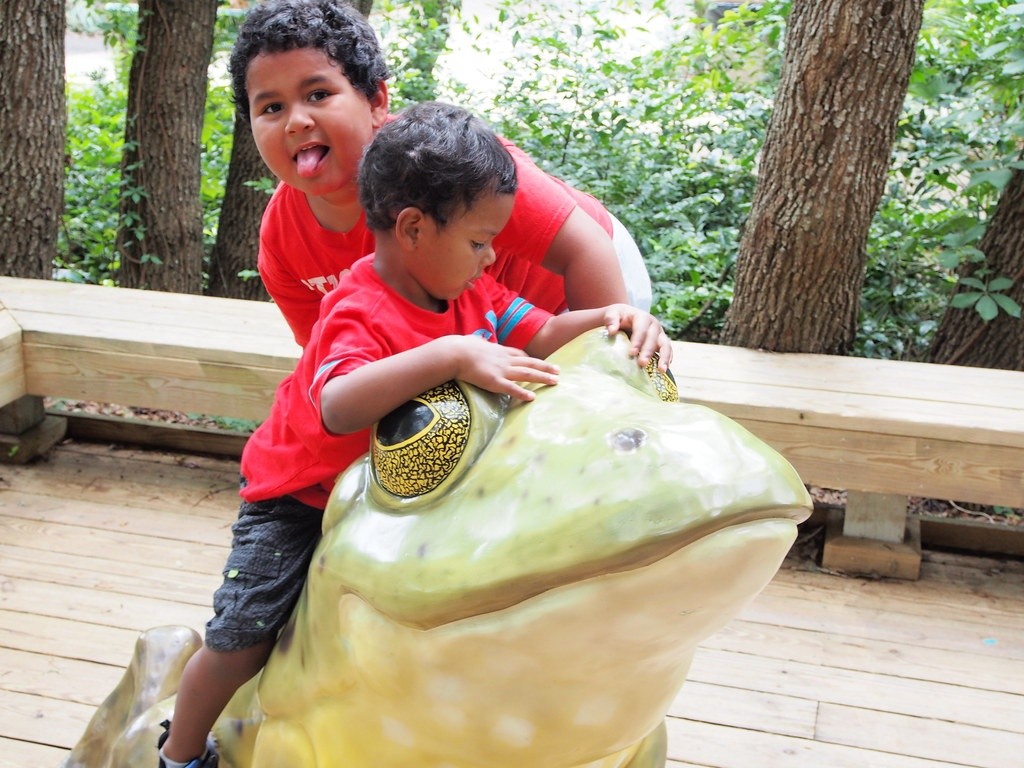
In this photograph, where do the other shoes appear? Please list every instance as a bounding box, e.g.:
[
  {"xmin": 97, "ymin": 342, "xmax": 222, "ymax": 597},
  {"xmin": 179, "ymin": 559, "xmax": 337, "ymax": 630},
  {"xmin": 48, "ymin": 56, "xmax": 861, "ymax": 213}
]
[{"xmin": 155, "ymin": 720, "xmax": 220, "ymax": 768}]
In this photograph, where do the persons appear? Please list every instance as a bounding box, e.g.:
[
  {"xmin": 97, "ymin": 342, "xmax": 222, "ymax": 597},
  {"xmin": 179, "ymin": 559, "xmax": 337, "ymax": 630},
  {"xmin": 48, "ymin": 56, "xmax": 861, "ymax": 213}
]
[
  {"xmin": 155, "ymin": 102, "xmax": 674, "ymax": 768},
  {"xmin": 230, "ymin": 1, "xmax": 655, "ymax": 351}
]
